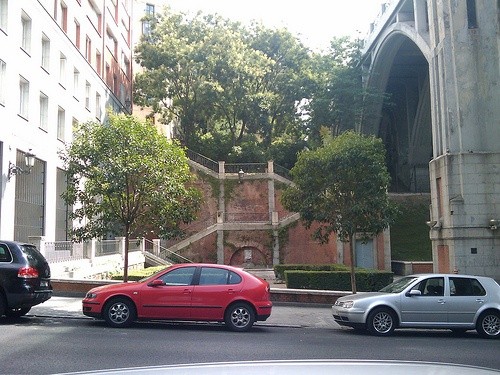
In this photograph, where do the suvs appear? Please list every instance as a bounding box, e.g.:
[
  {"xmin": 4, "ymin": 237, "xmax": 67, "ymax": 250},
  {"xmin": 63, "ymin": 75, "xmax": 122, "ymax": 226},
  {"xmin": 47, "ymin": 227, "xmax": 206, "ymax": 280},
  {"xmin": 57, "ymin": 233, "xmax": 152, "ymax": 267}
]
[{"xmin": 0, "ymin": 240, "xmax": 54, "ymax": 317}]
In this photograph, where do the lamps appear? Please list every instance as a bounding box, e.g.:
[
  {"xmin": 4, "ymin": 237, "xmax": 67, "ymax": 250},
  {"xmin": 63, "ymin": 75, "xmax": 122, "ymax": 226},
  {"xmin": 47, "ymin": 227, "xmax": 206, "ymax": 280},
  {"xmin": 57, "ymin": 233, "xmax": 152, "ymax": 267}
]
[{"xmin": 8, "ymin": 148, "xmax": 36, "ymax": 182}]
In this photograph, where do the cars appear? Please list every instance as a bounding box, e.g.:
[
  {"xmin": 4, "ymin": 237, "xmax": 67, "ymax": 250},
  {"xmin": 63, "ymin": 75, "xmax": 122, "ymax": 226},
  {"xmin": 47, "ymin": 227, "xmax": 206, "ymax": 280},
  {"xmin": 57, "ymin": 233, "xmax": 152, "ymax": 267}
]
[
  {"xmin": 81, "ymin": 263, "xmax": 273, "ymax": 331},
  {"xmin": 331, "ymin": 273, "xmax": 500, "ymax": 339}
]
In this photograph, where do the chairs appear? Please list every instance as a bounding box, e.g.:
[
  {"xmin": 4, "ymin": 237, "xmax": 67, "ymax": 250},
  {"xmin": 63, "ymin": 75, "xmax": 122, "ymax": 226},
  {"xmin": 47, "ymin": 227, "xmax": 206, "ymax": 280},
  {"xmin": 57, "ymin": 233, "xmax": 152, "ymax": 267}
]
[{"xmin": 427, "ymin": 285, "xmax": 481, "ymax": 294}]
